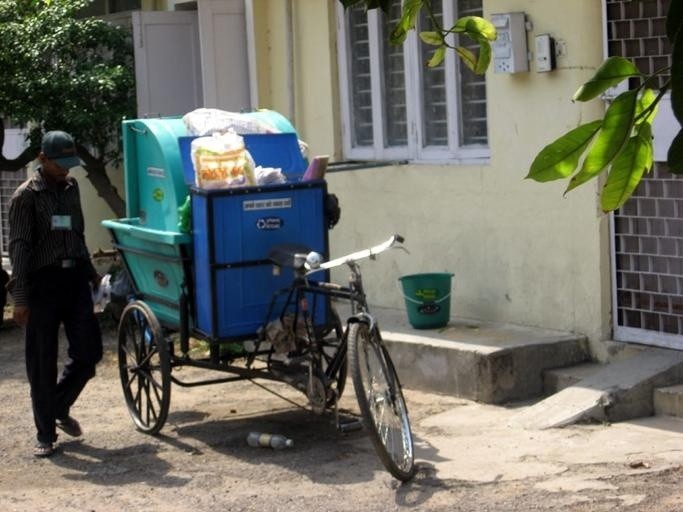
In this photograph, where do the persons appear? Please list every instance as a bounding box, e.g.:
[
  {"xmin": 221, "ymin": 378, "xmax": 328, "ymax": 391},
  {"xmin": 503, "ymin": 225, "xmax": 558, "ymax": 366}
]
[{"xmin": 9, "ymin": 130, "xmax": 107, "ymax": 460}]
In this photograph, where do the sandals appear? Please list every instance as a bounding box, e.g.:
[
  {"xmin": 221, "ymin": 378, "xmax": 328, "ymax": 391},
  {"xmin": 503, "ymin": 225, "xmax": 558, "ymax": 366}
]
[
  {"xmin": 54, "ymin": 416, "xmax": 80, "ymax": 436},
  {"xmin": 33, "ymin": 442, "xmax": 53, "ymax": 456}
]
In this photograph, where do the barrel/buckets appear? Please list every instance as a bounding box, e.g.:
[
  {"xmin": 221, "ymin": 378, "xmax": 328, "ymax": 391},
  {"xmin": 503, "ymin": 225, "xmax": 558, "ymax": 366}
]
[{"xmin": 395, "ymin": 272, "xmax": 455, "ymax": 330}]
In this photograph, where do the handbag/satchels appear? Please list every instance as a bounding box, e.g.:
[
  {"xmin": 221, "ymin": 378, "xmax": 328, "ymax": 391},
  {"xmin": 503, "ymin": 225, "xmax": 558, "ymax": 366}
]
[{"xmin": 265, "ymin": 311, "xmax": 321, "ymax": 368}]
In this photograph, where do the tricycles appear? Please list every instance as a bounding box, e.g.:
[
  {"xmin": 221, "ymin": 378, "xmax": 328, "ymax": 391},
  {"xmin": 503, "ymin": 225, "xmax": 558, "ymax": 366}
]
[{"xmin": 99, "ymin": 185, "xmax": 420, "ymax": 484}]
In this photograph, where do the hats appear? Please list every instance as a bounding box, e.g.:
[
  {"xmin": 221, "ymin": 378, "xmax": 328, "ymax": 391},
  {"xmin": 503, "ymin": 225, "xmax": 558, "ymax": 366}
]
[{"xmin": 41, "ymin": 130, "xmax": 80, "ymax": 168}]
